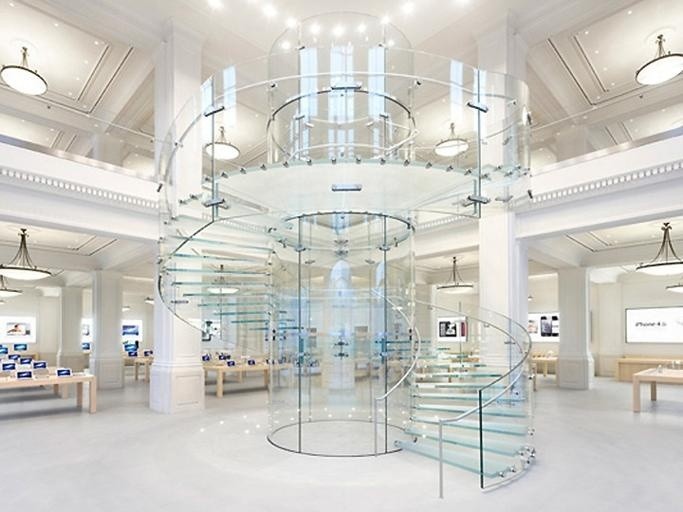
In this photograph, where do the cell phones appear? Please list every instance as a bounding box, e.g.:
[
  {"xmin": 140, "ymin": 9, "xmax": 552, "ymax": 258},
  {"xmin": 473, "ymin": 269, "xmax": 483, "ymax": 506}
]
[
  {"xmin": 439, "ymin": 320, "xmax": 465, "ymax": 337},
  {"xmin": 539, "ymin": 314, "xmax": 560, "ymax": 338}
]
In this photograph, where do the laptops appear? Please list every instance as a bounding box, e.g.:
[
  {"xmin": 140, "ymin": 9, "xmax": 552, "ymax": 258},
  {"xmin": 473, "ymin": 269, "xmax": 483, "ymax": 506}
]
[
  {"xmin": 125, "ymin": 344, "xmax": 137, "ymax": 352},
  {"xmin": 19, "ymin": 357, "xmax": 32, "ymax": 367},
  {"xmin": 0, "ymin": 348, "xmax": 9, "ymax": 358},
  {"xmin": 128, "ymin": 351, "xmax": 137, "ymax": 358},
  {"xmin": 13, "ymin": 343, "xmax": 28, "ymax": 352},
  {"xmin": 219, "ymin": 355, "xmax": 226, "ymax": 360},
  {"xmin": 225, "ymin": 355, "xmax": 231, "ymax": 360},
  {"xmin": 227, "ymin": 361, "xmax": 235, "ymax": 367},
  {"xmin": 82, "ymin": 343, "xmax": 90, "ymax": 350},
  {"xmin": 247, "ymin": 359, "xmax": 255, "ymax": 366},
  {"xmin": 263, "ymin": 354, "xmax": 320, "ymax": 367},
  {"xmin": 17, "ymin": 371, "xmax": 32, "ymax": 379},
  {"xmin": 8, "ymin": 353, "xmax": 22, "ymax": 363},
  {"xmin": 202, "ymin": 354, "xmax": 211, "ymax": 361},
  {"xmin": 143, "ymin": 350, "xmax": 153, "ymax": 357},
  {"xmin": 56, "ymin": 368, "xmax": 73, "ymax": 379},
  {"xmin": 32, "ymin": 361, "xmax": 49, "ymax": 378},
  {"xmin": 1, "ymin": 362, "xmax": 16, "ymax": 372}
]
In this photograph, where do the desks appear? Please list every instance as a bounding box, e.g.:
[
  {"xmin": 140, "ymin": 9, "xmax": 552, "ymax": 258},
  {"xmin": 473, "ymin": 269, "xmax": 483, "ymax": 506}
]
[
  {"xmin": 124, "ymin": 350, "xmax": 154, "ymax": 384},
  {"xmin": 203, "ymin": 355, "xmax": 293, "ymax": 398},
  {"xmin": 615, "ymin": 356, "xmax": 683, "ymax": 383},
  {"xmin": 633, "ymin": 368, "xmax": 683, "ymax": 412},
  {"xmin": 0, "ymin": 350, "xmax": 97, "ymax": 414}
]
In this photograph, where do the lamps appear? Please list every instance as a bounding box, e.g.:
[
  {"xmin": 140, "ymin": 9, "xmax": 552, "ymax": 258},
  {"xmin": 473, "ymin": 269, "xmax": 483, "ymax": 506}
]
[
  {"xmin": 204, "ymin": 126, "xmax": 240, "ymax": 161},
  {"xmin": 436, "ymin": 257, "xmax": 474, "ymax": 294},
  {"xmin": 635, "ymin": 25, "xmax": 683, "ymax": 86},
  {"xmin": 434, "ymin": 122, "xmax": 469, "ymax": 157},
  {"xmin": 207, "ymin": 265, "xmax": 239, "ymax": 294},
  {"xmin": 636, "ymin": 222, "xmax": 683, "ymax": 276},
  {"xmin": 666, "ymin": 285, "xmax": 683, "ymax": 294},
  {"xmin": 0, "ymin": 228, "xmax": 51, "ymax": 298},
  {"xmin": 0, "ymin": 39, "xmax": 48, "ymax": 96}
]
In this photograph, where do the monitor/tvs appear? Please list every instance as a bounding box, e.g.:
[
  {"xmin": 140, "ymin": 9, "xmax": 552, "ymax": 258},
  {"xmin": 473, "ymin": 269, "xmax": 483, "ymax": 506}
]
[
  {"xmin": 122, "ymin": 325, "xmax": 139, "ymax": 337},
  {"xmin": 6, "ymin": 322, "xmax": 31, "ymax": 336}
]
